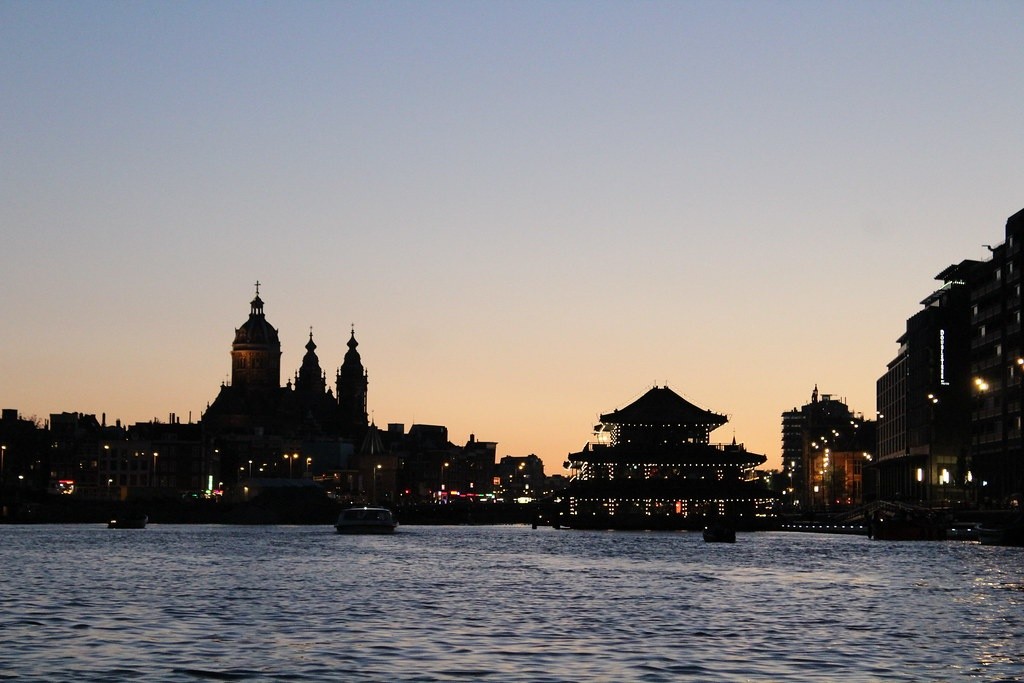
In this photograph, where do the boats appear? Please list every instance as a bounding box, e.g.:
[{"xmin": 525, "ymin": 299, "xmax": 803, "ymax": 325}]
[
  {"xmin": 108, "ymin": 514, "xmax": 149, "ymax": 529},
  {"xmin": 702, "ymin": 529, "xmax": 736, "ymax": 543},
  {"xmin": 334, "ymin": 506, "xmax": 397, "ymax": 535}
]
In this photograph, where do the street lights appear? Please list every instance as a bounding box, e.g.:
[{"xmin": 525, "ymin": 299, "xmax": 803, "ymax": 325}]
[
  {"xmin": 154, "ymin": 452, "xmax": 159, "ymax": 487},
  {"xmin": 441, "ymin": 462, "xmax": 450, "ymax": 487},
  {"xmin": 284, "ymin": 452, "xmax": 299, "ymax": 478},
  {"xmin": 373, "ymin": 464, "xmax": 382, "ymax": 500}
]
